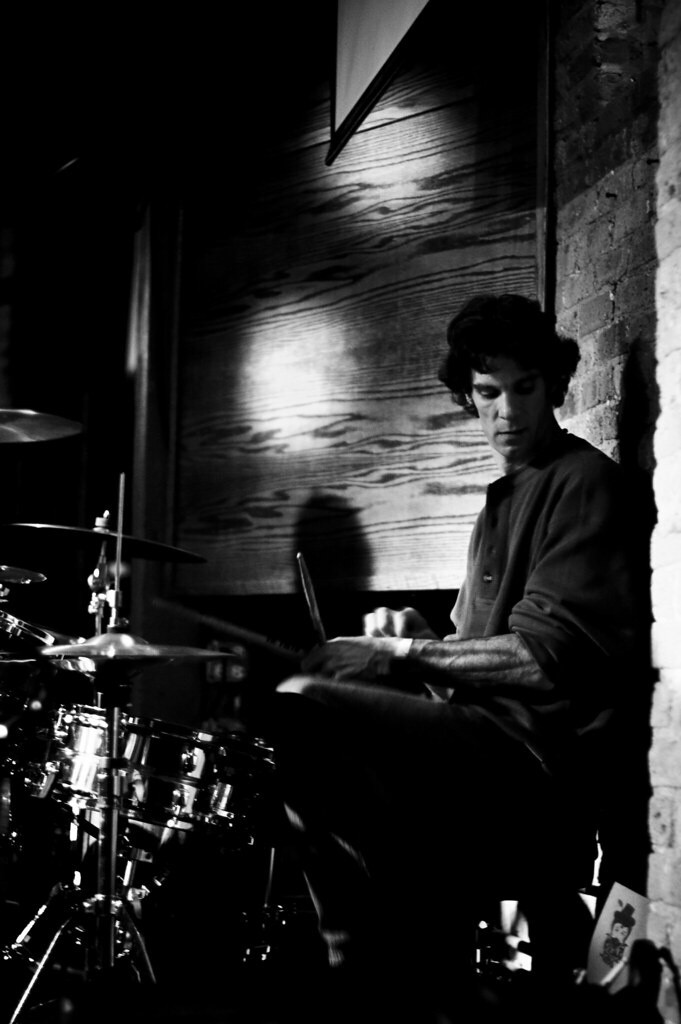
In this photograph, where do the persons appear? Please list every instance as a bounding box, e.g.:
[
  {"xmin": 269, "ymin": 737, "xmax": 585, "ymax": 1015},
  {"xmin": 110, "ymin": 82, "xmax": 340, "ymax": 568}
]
[{"xmin": 296, "ymin": 297, "xmax": 657, "ymax": 1024}]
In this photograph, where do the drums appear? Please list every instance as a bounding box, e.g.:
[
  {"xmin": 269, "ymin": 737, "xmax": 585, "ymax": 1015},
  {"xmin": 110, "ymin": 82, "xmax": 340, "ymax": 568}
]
[
  {"xmin": 50, "ymin": 701, "xmax": 147, "ymax": 813},
  {"xmin": 126, "ymin": 715, "xmax": 276, "ymax": 848}
]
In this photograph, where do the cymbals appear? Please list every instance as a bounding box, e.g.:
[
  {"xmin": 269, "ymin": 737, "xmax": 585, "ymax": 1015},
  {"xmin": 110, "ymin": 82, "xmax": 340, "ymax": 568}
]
[
  {"xmin": 10, "ymin": 506, "xmax": 210, "ymax": 567},
  {"xmin": 0, "ymin": 564, "xmax": 47, "ymax": 583},
  {"xmin": 1, "ymin": 630, "xmax": 241, "ymax": 664},
  {"xmin": 0, "ymin": 405, "xmax": 85, "ymax": 443}
]
